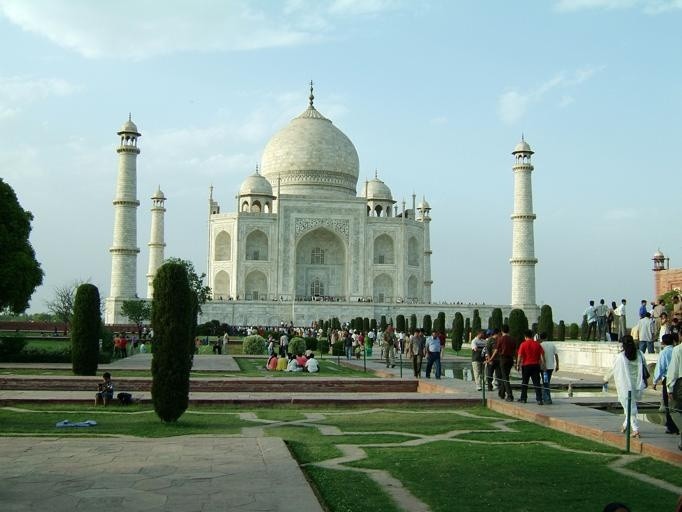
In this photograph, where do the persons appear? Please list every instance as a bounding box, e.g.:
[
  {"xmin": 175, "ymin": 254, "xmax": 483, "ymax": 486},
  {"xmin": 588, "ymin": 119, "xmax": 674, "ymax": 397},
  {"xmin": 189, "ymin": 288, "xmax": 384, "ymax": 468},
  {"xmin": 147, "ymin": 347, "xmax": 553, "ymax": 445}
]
[
  {"xmin": 52, "ymin": 326, "xmax": 57, "ymax": 334},
  {"xmin": 471, "ymin": 324, "xmax": 559, "ymax": 405},
  {"xmin": 112, "ymin": 324, "xmax": 154, "ymax": 360},
  {"xmin": 95, "ymin": 372, "xmax": 114, "ymax": 407},
  {"xmin": 196, "ymin": 321, "xmax": 445, "ymax": 380},
  {"xmin": 583, "ymin": 296, "xmax": 682, "ymax": 451}
]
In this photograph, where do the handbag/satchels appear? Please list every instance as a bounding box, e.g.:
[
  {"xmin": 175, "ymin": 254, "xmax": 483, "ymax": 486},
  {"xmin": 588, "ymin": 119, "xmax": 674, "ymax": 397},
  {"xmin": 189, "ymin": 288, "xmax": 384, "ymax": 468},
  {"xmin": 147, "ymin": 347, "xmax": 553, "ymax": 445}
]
[{"xmin": 643, "ymin": 364, "xmax": 650, "ymax": 379}]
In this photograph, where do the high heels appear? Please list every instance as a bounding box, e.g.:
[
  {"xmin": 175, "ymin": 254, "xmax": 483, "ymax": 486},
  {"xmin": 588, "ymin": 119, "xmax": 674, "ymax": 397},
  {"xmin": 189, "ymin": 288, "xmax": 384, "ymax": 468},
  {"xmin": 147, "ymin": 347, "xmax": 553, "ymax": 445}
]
[{"xmin": 630, "ymin": 431, "xmax": 640, "ymax": 439}]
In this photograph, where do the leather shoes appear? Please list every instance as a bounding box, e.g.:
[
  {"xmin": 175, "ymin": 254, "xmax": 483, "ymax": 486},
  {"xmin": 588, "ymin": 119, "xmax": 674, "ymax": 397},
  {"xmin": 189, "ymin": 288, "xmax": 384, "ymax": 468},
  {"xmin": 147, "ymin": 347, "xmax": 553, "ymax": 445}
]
[{"xmin": 498, "ymin": 393, "xmax": 552, "ymax": 405}]
